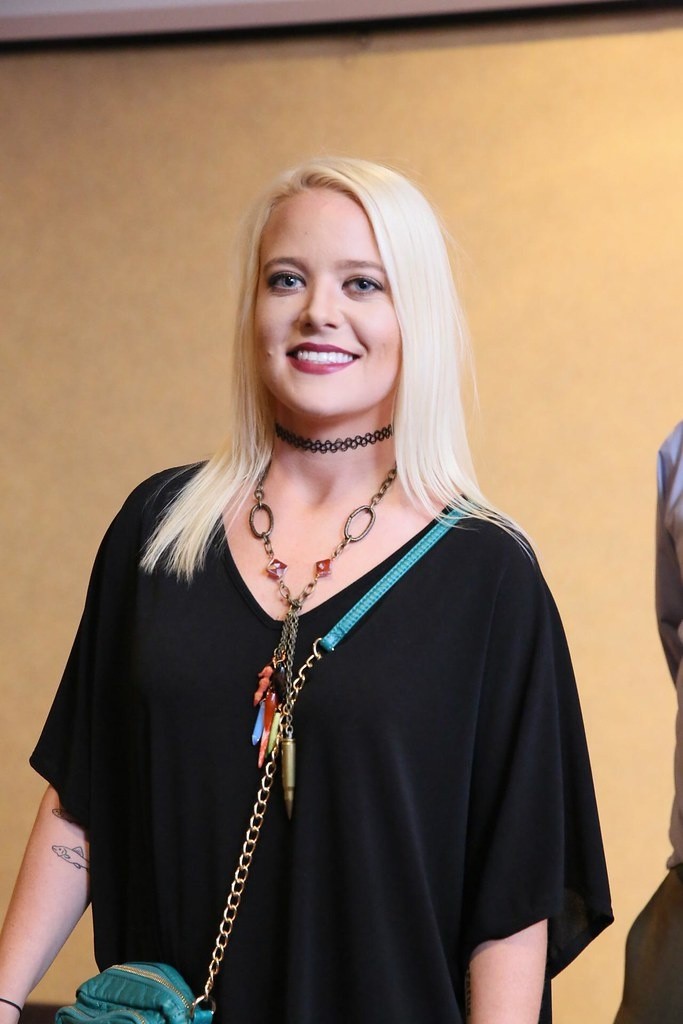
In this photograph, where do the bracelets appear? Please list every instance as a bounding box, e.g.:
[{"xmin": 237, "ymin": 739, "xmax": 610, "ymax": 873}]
[{"xmin": 0, "ymin": 998, "xmax": 22, "ymax": 1017}]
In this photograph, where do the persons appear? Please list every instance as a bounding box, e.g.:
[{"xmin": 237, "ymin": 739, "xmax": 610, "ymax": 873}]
[
  {"xmin": 0, "ymin": 159, "xmax": 615, "ymax": 1024},
  {"xmin": 612, "ymin": 418, "xmax": 682, "ymax": 1024}
]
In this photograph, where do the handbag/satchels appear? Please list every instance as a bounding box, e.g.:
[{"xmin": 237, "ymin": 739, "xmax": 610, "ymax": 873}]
[{"xmin": 55, "ymin": 961, "xmax": 217, "ymax": 1024}]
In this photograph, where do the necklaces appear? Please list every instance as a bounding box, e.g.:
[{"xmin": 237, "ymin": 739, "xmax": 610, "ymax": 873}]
[
  {"xmin": 252, "ymin": 466, "xmax": 397, "ymax": 817},
  {"xmin": 272, "ymin": 426, "xmax": 394, "ymax": 454}
]
